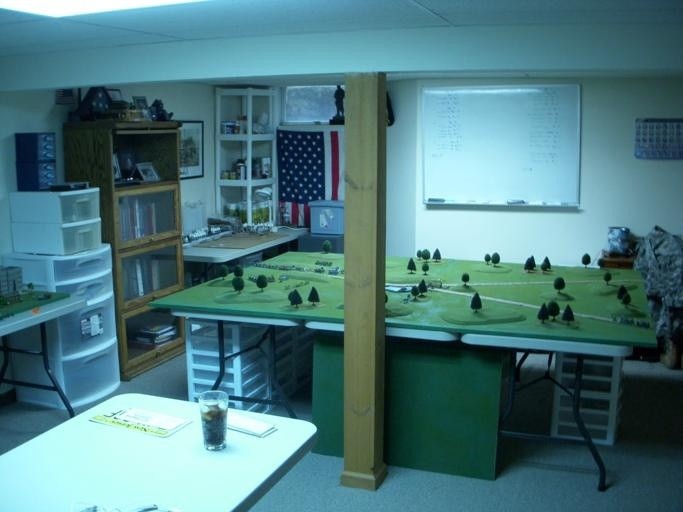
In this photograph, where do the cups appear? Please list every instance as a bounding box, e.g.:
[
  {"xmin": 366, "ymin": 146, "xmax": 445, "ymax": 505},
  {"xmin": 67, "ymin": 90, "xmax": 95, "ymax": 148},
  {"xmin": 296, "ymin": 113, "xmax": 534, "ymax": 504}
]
[{"xmin": 198, "ymin": 389, "xmax": 230, "ymax": 452}]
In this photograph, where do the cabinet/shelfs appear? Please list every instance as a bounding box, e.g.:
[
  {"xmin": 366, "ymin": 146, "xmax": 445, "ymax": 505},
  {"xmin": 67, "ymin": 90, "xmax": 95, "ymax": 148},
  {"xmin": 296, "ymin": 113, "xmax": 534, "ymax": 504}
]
[
  {"xmin": 65, "ymin": 121, "xmax": 187, "ymax": 378},
  {"xmin": 214, "ymin": 88, "xmax": 281, "ymax": 227}
]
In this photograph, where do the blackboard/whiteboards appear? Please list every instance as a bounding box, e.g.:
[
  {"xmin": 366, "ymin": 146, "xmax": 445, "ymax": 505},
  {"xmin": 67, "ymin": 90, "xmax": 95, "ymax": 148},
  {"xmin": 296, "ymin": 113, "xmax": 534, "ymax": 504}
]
[{"xmin": 417, "ymin": 82, "xmax": 584, "ymax": 210}]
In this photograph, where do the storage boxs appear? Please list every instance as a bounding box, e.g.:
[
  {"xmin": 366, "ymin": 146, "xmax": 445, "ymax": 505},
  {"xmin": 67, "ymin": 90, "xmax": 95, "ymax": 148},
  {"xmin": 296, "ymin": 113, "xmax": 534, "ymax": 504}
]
[
  {"xmin": 7, "ymin": 132, "xmax": 120, "ymax": 410},
  {"xmin": 308, "ymin": 201, "xmax": 344, "ymax": 236}
]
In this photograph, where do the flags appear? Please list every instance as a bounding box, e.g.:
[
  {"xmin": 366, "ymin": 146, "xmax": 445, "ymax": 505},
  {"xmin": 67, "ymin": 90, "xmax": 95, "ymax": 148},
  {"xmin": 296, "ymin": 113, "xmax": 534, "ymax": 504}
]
[{"xmin": 275, "ymin": 125, "xmax": 345, "ymax": 227}]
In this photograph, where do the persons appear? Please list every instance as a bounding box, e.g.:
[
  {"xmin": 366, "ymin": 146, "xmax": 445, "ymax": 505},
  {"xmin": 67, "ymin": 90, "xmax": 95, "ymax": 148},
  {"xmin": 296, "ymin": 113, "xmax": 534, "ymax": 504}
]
[{"xmin": 331, "ymin": 83, "xmax": 345, "ymax": 117}]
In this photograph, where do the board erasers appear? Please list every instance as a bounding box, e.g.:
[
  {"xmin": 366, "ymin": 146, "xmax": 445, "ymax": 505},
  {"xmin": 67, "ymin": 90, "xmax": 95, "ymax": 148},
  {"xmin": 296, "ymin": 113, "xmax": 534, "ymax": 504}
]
[
  {"xmin": 508, "ymin": 199, "xmax": 525, "ymax": 205},
  {"xmin": 428, "ymin": 198, "xmax": 445, "ymax": 202}
]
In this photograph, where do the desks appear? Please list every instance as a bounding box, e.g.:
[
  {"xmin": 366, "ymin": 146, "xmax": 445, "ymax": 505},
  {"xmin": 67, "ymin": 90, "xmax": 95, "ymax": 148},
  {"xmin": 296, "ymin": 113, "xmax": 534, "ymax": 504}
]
[
  {"xmin": 2, "ymin": 394, "xmax": 318, "ymax": 512},
  {"xmin": 181, "ymin": 221, "xmax": 297, "ymax": 282},
  {"xmin": 0, "ymin": 290, "xmax": 87, "ymax": 419}
]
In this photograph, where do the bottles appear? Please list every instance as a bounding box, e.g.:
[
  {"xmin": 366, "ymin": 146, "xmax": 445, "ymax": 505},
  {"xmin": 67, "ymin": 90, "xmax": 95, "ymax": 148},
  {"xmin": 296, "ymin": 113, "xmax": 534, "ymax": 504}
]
[
  {"xmin": 233, "ymin": 120, "xmax": 240, "ymax": 135},
  {"xmin": 220, "ymin": 159, "xmax": 263, "ymax": 180}
]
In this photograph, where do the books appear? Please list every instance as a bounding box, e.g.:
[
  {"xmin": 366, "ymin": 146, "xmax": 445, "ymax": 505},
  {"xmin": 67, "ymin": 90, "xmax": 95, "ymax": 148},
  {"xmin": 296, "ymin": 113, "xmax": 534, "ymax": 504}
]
[{"xmin": 117, "ymin": 196, "xmax": 178, "ymax": 352}]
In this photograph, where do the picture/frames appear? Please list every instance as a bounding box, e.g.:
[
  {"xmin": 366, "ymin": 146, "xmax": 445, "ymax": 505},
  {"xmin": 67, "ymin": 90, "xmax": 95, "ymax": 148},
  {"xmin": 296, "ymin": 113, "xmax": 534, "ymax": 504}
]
[
  {"xmin": 133, "ymin": 96, "xmax": 147, "ymax": 112},
  {"xmin": 174, "ymin": 119, "xmax": 205, "ymax": 180},
  {"xmin": 129, "ymin": 162, "xmax": 161, "ymax": 183}
]
